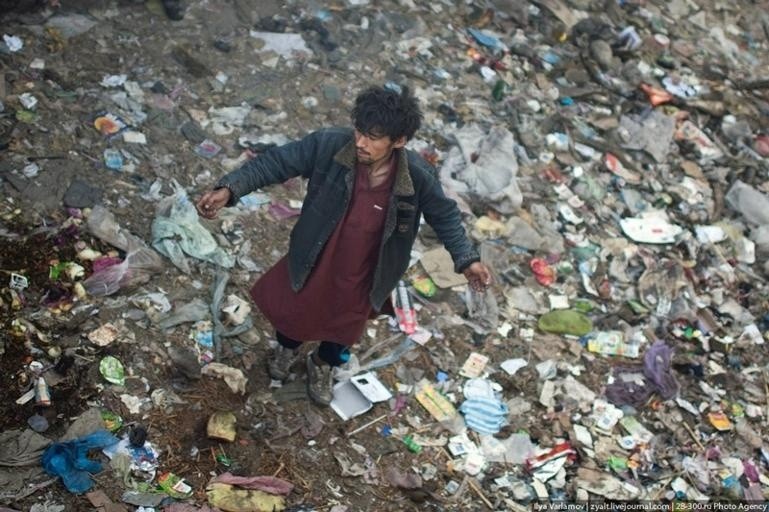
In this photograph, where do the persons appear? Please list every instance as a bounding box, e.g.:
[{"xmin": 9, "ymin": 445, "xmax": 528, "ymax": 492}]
[{"xmin": 195, "ymin": 85, "xmax": 493, "ymax": 405}]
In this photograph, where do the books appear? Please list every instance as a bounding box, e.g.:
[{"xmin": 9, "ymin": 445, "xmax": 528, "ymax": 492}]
[{"xmin": 330, "ymin": 371, "xmax": 392, "ymax": 420}]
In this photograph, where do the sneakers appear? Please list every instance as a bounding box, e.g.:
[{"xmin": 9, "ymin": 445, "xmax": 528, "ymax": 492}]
[
  {"xmin": 307, "ymin": 350, "xmax": 334, "ymax": 405},
  {"xmin": 270, "ymin": 345, "xmax": 299, "ymax": 380}
]
